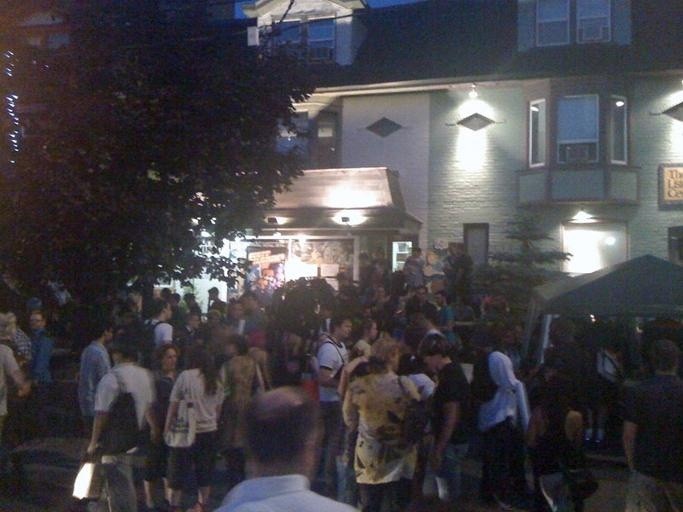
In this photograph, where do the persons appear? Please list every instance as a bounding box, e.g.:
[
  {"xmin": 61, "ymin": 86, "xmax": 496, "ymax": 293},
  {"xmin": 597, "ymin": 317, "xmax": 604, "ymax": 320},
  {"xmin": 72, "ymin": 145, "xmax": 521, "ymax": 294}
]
[{"xmin": 1, "ymin": 243, "xmax": 683, "ymax": 511}]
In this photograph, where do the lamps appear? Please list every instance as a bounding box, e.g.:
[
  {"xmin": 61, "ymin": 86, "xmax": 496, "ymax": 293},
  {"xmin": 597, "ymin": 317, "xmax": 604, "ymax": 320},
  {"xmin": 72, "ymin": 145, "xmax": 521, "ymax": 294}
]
[
  {"xmin": 341, "ymin": 216, "xmax": 350, "ymax": 223},
  {"xmin": 267, "ymin": 217, "xmax": 278, "ymax": 227},
  {"xmin": 468, "ymin": 85, "xmax": 478, "ymax": 99}
]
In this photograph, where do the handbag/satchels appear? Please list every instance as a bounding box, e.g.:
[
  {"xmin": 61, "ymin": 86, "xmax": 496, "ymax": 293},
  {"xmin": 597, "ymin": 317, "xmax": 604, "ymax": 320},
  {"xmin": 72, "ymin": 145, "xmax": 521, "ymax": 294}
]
[
  {"xmin": 164, "ymin": 401, "xmax": 196, "ymax": 449},
  {"xmin": 73, "ymin": 463, "xmax": 100, "ymax": 502},
  {"xmin": 98, "ymin": 393, "xmax": 136, "ymax": 453},
  {"xmin": 400, "ymin": 400, "xmax": 426, "ymax": 441}
]
